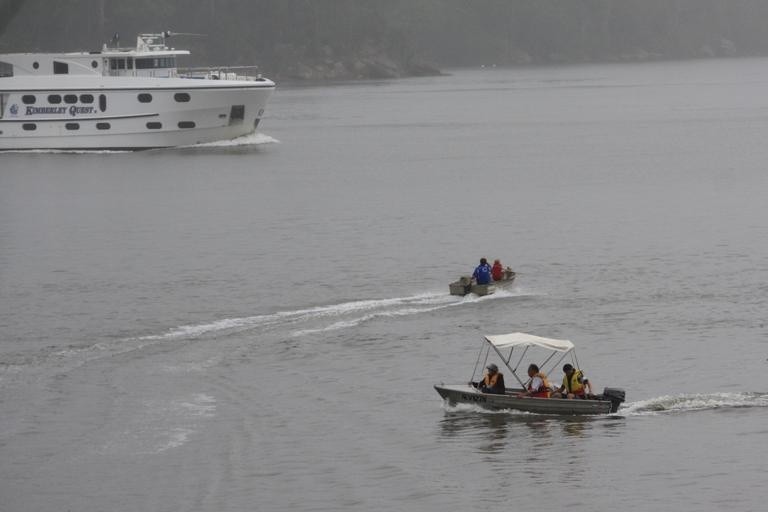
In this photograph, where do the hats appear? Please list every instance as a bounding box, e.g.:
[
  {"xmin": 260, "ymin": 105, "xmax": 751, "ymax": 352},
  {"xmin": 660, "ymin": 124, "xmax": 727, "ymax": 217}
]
[{"xmin": 487, "ymin": 364, "xmax": 498, "ymax": 372}]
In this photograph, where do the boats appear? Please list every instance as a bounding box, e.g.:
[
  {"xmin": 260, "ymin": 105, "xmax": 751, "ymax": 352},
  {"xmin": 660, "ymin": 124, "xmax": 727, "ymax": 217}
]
[
  {"xmin": 448, "ymin": 271, "xmax": 516, "ymax": 297},
  {"xmin": 0, "ymin": 31, "xmax": 277, "ymax": 153},
  {"xmin": 434, "ymin": 382, "xmax": 627, "ymax": 415}
]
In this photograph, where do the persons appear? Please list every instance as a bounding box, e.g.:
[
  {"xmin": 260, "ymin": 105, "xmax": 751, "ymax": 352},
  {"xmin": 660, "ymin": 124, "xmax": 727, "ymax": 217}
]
[
  {"xmin": 468, "ymin": 363, "xmax": 505, "ymax": 396},
  {"xmin": 490, "ymin": 259, "xmax": 503, "ymax": 281},
  {"xmin": 515, "ymin": 362, "xmax": 549, "ymax": 401},
  {"xmin": 470, "ymin": 258, "xmax": 492, "ymax": 285},
  {"xmin": 549, "ymin": 364, "xmax": 594, "ymax": 399}
]
